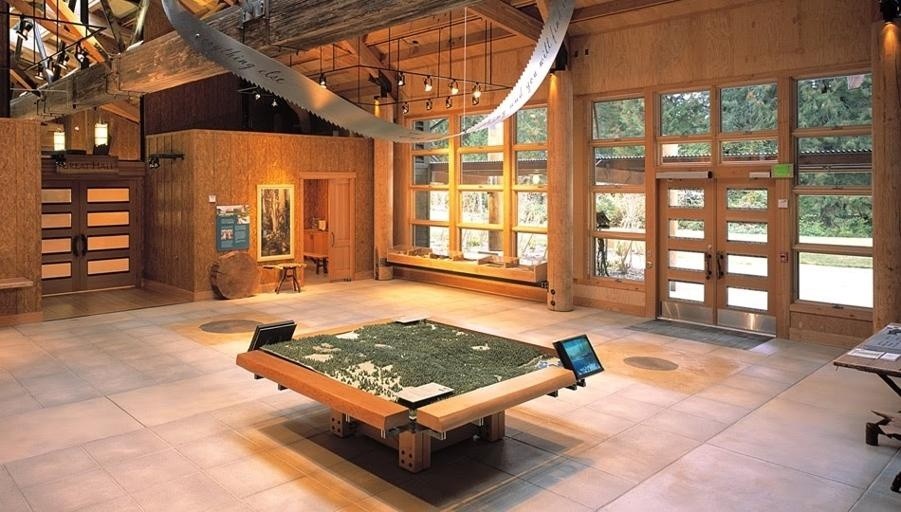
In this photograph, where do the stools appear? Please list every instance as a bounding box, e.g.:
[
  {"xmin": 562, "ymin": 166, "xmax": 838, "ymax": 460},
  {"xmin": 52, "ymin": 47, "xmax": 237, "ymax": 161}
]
[
  {"xmin": 262, "ymin": 263, "xmax": 307, "ymax": 294},
  {"xmin": 304, "ymin": 252, "xmax": 328, "ymax": 275}
]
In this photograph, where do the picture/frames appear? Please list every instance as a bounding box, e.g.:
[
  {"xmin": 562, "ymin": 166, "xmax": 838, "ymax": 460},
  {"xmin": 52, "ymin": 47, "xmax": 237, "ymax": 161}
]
[{"xmin": 256, "ymin": 184, "xmax": 295, "ymax": 263}]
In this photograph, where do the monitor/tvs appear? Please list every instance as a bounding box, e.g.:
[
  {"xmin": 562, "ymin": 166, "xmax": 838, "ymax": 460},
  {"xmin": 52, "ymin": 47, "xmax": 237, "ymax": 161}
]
[
  {"xmin": 246, "ymin": 320, "xmax": 297, "ymax": 351},
  {"xmin": 554, "ymin": 334, "xmax": 604, "ymax": 380}
]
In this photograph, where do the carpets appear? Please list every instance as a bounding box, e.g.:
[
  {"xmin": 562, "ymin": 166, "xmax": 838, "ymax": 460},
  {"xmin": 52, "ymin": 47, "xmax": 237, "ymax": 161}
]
[{"xmin": 624, "ymin": 319, "xmax": 775, "ymax": 351}]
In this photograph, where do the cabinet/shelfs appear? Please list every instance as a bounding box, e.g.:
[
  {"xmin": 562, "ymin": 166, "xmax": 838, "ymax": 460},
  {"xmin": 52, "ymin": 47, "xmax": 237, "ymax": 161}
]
[{"xmin": 304, "ymin": 228, "xmax": 328, "ymax": 254}]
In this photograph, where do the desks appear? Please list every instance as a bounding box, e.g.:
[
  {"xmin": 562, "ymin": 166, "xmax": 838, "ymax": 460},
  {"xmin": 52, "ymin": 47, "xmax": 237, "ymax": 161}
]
[
  {"xmin": 236, "ymin": 313, "xmax": 605, "ymax": 474},
  {"xmin": 387, "ymin": 243, "xmax": 547, "ymax": 304},
  {"xmin": 833, "ymin": 322, "xmax": 901, "ymax": 493}
]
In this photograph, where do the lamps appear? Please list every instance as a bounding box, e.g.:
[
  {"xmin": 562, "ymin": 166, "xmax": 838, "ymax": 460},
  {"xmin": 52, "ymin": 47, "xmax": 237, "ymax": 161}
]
[
  {"xmin": 94, "ymin": 105, "xmax": 108, "ymax": 147},
  {"xmin": 0, "ymin": 0, "xmax": 107, "ymax": 81},
  {"xmin": 149, "ymin": 153, "xmax": 184, "ymax": 169},
  {"xmin": 53, "ymin": 118, "xmax": 65, "ymax": 151},
  {"xmin": 235, "ymin": 10, "xmax": 513, "ymax": 114}
]
